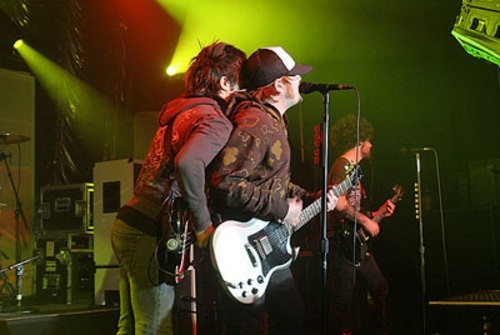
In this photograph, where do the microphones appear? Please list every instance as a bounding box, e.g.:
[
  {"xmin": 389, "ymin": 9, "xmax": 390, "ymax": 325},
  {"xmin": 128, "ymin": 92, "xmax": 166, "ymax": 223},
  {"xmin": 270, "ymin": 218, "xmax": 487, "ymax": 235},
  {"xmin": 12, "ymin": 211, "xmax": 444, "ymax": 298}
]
[
  {"xmin": 400, "ymin": 147, "xmax": 433, "ymax": 155},
  {"xmin": 299, "ymin": 82, "xmax": 353, "ymax": 95}
]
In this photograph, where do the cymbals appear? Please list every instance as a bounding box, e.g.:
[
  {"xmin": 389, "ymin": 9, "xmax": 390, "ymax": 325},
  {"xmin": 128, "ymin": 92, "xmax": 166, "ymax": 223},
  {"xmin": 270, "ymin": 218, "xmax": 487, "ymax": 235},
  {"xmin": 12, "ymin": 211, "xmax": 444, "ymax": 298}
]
[{"xmin": 0, "ymin": 133, "xmax": 31, "ymax": 145}]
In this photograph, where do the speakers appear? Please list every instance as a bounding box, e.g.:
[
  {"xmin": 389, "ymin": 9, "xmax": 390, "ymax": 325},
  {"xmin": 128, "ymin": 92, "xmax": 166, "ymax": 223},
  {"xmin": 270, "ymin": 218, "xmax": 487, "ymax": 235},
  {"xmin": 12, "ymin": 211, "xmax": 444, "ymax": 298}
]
[{"xmin": 92, "ymin": 159, "xmax": 144, "ymax": 268}]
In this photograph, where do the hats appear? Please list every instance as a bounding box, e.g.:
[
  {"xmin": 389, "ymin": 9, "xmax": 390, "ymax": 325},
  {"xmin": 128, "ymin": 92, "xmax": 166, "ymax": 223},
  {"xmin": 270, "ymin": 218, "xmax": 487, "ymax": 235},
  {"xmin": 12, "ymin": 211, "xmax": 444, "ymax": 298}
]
[{"xmin": 244, "ymin": 46, "xmax": 312, "ymax": 91}]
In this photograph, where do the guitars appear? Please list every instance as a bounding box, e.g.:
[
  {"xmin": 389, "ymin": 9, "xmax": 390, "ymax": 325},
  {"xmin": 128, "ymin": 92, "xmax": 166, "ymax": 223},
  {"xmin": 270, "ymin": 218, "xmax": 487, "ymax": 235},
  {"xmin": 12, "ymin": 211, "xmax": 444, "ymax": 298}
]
[
  {"xmin": 337, "ymin": 184, "xmax": 404, "ymax": 269},
  {"xmin": 209, "ymin": 160, "xmax": 364, "ymax": 305},
  {"xmin": 165, "ymin": 197, "xmax": 223, "ymax": 286}
]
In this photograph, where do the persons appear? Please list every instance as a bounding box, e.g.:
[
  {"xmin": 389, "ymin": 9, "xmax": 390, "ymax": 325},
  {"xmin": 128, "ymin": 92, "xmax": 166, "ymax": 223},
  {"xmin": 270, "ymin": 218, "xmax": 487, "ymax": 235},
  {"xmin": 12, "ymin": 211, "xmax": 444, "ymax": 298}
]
[
  {"xmin": 112, "ymin": 43, "xmax": 246, "ymax": 335},
  {"xmin": 207, "ymin": 46, "xmax": 339, "ymax": 335},
  {"xmin": 327, "ymin": 111, "xmax": 397, "ymax": 335}
]
